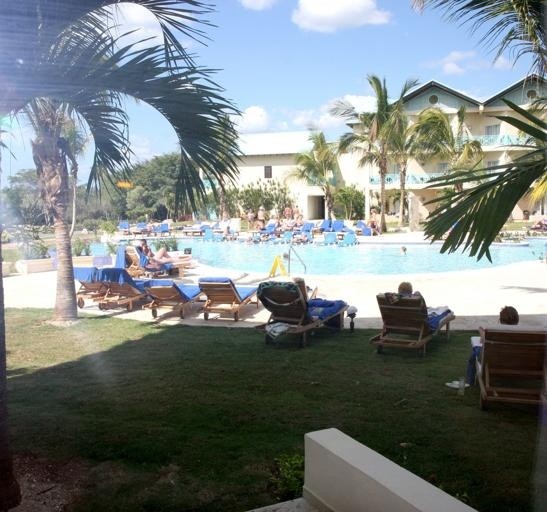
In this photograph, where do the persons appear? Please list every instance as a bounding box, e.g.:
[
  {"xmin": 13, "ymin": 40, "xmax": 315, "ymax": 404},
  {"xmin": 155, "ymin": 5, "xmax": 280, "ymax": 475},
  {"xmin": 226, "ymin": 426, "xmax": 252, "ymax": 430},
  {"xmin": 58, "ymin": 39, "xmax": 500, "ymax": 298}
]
[
  {"xmin": 292, "ymin": 214, "xmax": 305, "ymax": 227},
  {"xmin": 256, "ymin": 205, "xmax": 267, "ymax": 230},
  {"xmin": 293, "ymin": 205, "xmax": 301, "ymax": 220},
  {"xmin": 224, "ymin": 226, "xmax": 231, "ymax": 236},
  {"xmin": 291, "ymin": 276, "xmax": 309, "ymax": 312},
  {"xmin": 367, "ymin": 209, "xmax": 381, "ymax": 239},
  {"xmin": 479, "ymin": 305, "xmax": 520, "ymax": 344},
  {"xmin": 400, "ymin": 246, "xmax": 408, "ymax": 256},
  {"xmin": 397, "ymin": 281, "xmax": 427, "ymax": 310},
  {"xmin": 247, "ymin": 208, "xmax": 256, "ymax": 230},
  {"xmin": 133, "ymin": 238, "xmax": 193, "ymax": 267},
  {"xmin": 282, "ymin": 204, "xmax": 292, "ymax": 219},
  {"xmin": 272, "ymin": 205, "xmax": 279, "ymax": 219}
]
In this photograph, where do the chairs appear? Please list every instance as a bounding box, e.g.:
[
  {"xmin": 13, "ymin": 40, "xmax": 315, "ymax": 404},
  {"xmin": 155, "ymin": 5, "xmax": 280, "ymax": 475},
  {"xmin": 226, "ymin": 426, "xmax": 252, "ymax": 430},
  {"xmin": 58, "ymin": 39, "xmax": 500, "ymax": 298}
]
[
  {"xmin": 72, "ymin": 219, "xmax": 239, "ymax": 277},
  {"xmin": 244, "ymin": 219, "xmax": 371, "ymax": 248},
  {"xmin": 470, "ymin": 324, "xmax": 547, "ymax": 410},
  {"xmin": 197, "ymin": 277, "xmax": 258, "ymax": 322},
  {"xmin": 370, "ymin": 296, "xmax": 455, "ymax": 357},
  {"xmin": 142, "ymin": 279, "xmax": 201, "ymax": 320},
  {"xmin": 72, "ymin": 266, "xmax": 104, "ymax": 309},
  {"xmin": 256, "ymin": 281, "xmax": 350, "ymax": 344},
  {"xmin": 98, "ymin": 268, "xmax": 148, "ymax": 313}
]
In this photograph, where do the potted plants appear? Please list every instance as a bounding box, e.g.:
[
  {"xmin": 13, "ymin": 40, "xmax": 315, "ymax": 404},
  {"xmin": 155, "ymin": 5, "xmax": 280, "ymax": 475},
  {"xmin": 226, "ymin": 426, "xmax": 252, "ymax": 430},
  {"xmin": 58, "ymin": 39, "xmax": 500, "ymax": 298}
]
[
  {"xmin": 2, "ymin": 255, "xmax": 12, "ymax": 277},
  {"xmin": 15, "ymin": 245, "xmax": 53, "ymax": 274}
]
